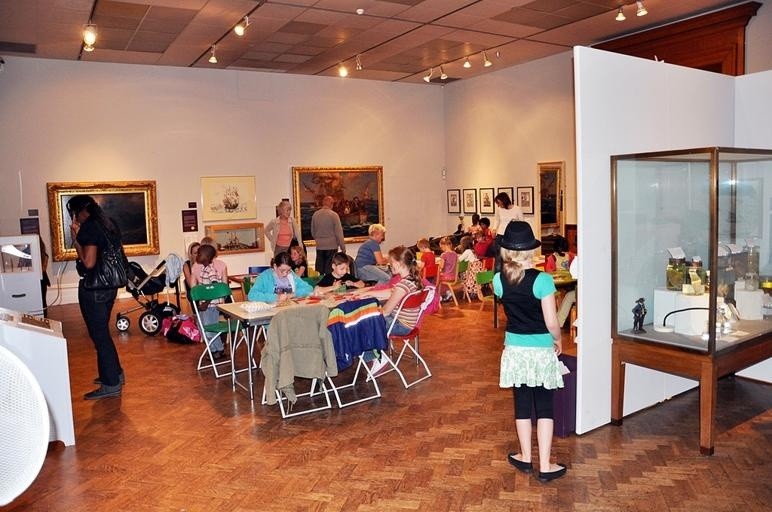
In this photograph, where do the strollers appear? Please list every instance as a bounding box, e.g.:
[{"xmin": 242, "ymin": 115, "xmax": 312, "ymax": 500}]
[{"xmin": 115, "ymin": 259, "xmax": 178, "ymax": 335}]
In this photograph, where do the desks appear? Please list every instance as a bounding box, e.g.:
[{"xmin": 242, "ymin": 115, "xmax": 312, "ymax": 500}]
[
  {"xmin": 611, "ymin": 315, "xmax": 772, "ymax": 456},
  {"xmin": 215, "ymin": 286, "xmax": 418, "ymax": 400}
]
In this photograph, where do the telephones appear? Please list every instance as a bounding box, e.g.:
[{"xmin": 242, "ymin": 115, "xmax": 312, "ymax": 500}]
[{"xmin": 71, "ymin": 213, "xmax": 78, "ymax": 234}]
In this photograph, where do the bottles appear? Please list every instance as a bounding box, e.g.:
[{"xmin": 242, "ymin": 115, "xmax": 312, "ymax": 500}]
[
  {"xmin": 706, "ymin": 242, "xmax": 763, "ymax": 281},
  {"xmin": 686, "ymin": 259, "xmax": 706, "ymax": 286},
  {"xmin": 665, "ymin": 258, "xmax": 687, "ymax": 291},
  {"xmin": 544, "ymin": 253, "xmax": 556, "ymax": 273},
  {"xmin": 760, "ymin": 282, "xmax": 772, "ymax": 319}
]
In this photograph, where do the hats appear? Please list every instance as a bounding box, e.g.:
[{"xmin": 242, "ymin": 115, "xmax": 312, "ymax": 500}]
[{"xmin": 495, "ymin": 221, "xmax": 541, "ymax": 251}]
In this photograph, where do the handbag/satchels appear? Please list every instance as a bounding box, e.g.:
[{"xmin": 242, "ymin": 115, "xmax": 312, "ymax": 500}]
[
  {"xmin": 166, "ymin": 315, "xmax": 200, "ymax": 344},
  {"xmin": 84, "ymin": 251, "xmax": 128, "ymax": 287}
]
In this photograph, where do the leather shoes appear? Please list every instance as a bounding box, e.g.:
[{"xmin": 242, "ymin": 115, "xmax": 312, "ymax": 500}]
[
  {"xmin": 94, "ymin": 368, "xmax": 126, "ymax": 385},
  {"xmin": 85, "ymin": 383, "xmax": 122, "ymax": 399}
]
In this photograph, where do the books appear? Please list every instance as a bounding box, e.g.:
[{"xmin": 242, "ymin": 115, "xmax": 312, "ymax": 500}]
[{"xmin": 556, "ymin": 291, "xmax": 577, "ymax": 344}]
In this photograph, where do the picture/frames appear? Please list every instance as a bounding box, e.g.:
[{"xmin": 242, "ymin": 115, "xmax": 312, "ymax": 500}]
[
  {"xmin": 47, "ymin": 179, "xmax": 161, "ymax": 262},
  {"xmin": 497, "ymin": 187, "xmax": 514, "ymax": 204},
  {"xmin": 292, "ymin": 165, "xmax": 386, "ymax": 247},
  {"xmin": 205, "ymin": 223, "xmax": 265, "ymax": 256},
  {"xmin": 463, "ymin": 188, "xmax": 477, "ymax": 213},
  {"xmin": 447, "ymin": 189, "xmax": 460, "ymax": 213},
  {"xmin": 517, "ymin": 186, "xmax": 534, "ymax": 214},
  {"xmin": 479, "ymin": 188, "xmax": 496, "ymax": 214}
]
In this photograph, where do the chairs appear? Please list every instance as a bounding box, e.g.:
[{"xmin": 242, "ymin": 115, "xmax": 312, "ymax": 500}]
[
  {"xmin": 184, "ymin": 252, "xmax": 578, "ymax": 344},
  {"xmin": 364, "ymin": 290, "xmax": 434, "ymax": 389},
  {"xmin": 188, "ymin": 283, "xmax": 256, "ymax": 379},
  {"xmin": 311, "ymin": 299, "xmax": 382, "ymax": 408},
  {"xmin": 261, "ymin": 305, "xmax": 331, "ymax": 418}
]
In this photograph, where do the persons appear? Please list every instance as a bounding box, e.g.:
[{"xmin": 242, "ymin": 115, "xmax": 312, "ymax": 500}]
[
  {"xmin": 417, "ymin": 213, "xmax": 495, "ymax": 302},
  {"xmin": 631, "ymin": 297, "xmax": 648, "ymax": 334},
  {"xmin": 67, "ymin": 195, "xmax": 128, "ymax": 399},
  {"xmin": 17, "ymin": 232, "xmax": 52, "ymax": 319},
  {"xmin": 182, "ymin": 237, "xmax": 229, "ymax": 359},
  {"xmin": 249, "ymin": 196, "xmax": 424, "ymax": 377},
  {"xmin": 491, "ymin": 191, "xmax": 523, "ymax": 257},
  {"xmin": 491, "ymin": 221, "xmax": 566, "ymax": 482}
]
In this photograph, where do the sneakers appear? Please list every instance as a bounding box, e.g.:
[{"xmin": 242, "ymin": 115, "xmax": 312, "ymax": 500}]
[
  {"xmin": 206, "ymin": 349, "xmax": 226, "ymax": 360},
  {"xmin": 444, "ymin": 293, "xmax": 452, "ymax": 301},
  {"xmin": 507, "ymin": 451, "xmax": 534, "ymax": 473},
  {"xmin": 539, "ymin": 463, "xmax": 566, "ymax": 481},
  {"xmin": 367, "ymin": 354, "xmax": 388, "ymax": 376}
]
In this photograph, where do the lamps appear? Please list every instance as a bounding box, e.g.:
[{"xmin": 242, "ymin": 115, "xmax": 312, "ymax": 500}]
[
  {"xmin": 481, "ymin": 48, "xmax": 494, "ymax": 68},
  {"xmin": 423, "ymin": 68, "xmax": 433, "ymax": 82},
  {"xmin": 81, "ymin": 20, "xmax": 99, "ymax": 52},
  {"xmin": 208, "ymin": 43, "xmax": 219, "ymax": 64},
  {"xmin": 339, "ymin": 63, "xmax": 348, "ymax": 77},
  {"xmin": 615, "ymin": 8, "xmax": 626, "ymax": 21},
  {"xmin": 437, "ymin": 65, "xmax": 448, "ymax": 80},
  {"xmin": 635, "ymin": 0, "xmax": 649, "ymax": 17},
  {"xmin": 463, "ymin": 58, "xmax": 472, "ymax": 68},
  {"xmin": 234, "ymin": 16, "xmax": 250, "ymax": 36}
]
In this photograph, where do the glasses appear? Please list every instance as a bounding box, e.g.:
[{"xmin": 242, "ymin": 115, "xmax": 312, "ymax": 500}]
[{"xmin": 278, "ymin": 268, "xmax": 292, "ymax": 274}]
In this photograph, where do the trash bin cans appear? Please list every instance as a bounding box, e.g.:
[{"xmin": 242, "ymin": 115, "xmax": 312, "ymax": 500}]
[{"xmin": 530, "ymin": 353, "xmax": 577, "ymax": 439}]
